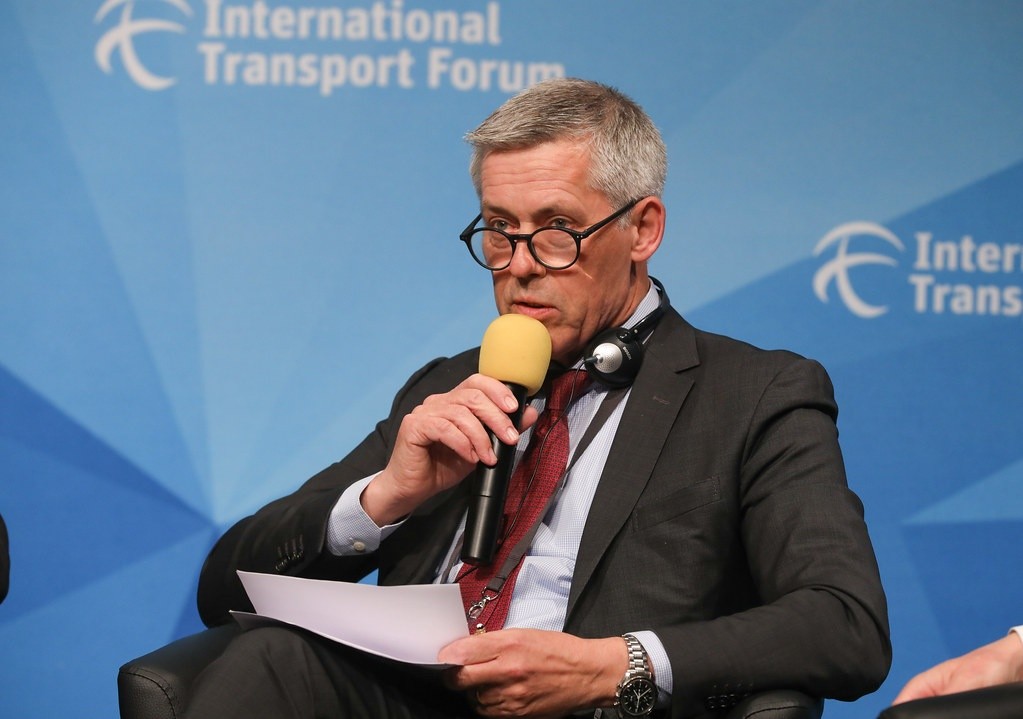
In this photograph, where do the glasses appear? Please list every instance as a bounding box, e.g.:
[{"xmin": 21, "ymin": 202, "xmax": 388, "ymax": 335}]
[{"xmin": 459, "ymin": 196, "xmax": 644, "ymax": 271}]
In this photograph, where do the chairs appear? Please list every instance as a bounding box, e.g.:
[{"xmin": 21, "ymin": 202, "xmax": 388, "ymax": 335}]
[{"xmin": 118, "ymin": 623, "xmax": 824, "ymax": 719}]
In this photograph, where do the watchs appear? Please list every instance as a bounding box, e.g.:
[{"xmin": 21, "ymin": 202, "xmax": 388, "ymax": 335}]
[{"xmin": 613, "ymin": 636, "xmax": 658, "ymax": 719}]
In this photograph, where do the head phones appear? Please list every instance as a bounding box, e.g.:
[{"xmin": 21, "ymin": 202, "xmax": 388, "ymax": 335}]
[{"xmin": 583, "ymin": 272, "xmax": 671, "ymax": 388}]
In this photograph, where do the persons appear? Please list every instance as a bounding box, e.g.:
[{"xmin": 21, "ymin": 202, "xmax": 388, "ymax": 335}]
[
  {"xmin": 187, "ymin": 78, "xmax": 892, "ymax": 719},
  {"xmin": 892, "ymin": 625, "xmax": 1023, "ymax": 705}
]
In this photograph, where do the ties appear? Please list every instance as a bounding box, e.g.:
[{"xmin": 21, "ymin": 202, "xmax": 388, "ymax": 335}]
[{"xmin": 453, "ymin": 367, "xmax": 596, "ymax": 636}]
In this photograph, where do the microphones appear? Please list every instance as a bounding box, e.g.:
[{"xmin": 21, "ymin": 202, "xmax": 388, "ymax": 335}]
[{"xmin": 459, "ymin": 313, "xmax": 552, "ymax": 568}]
